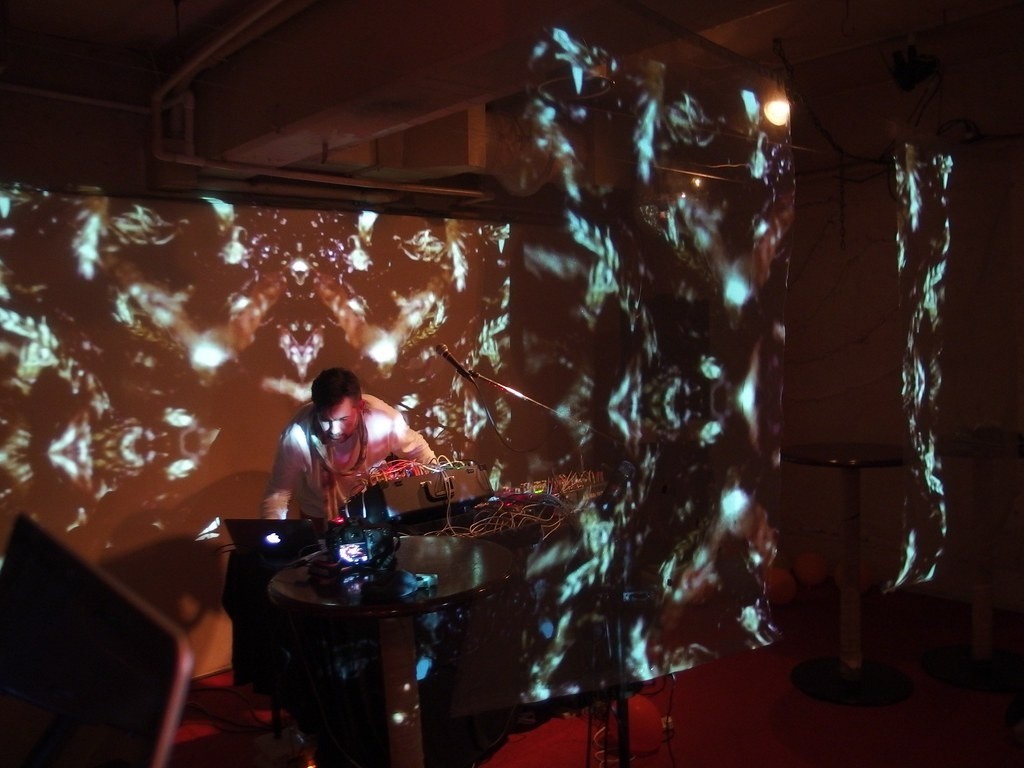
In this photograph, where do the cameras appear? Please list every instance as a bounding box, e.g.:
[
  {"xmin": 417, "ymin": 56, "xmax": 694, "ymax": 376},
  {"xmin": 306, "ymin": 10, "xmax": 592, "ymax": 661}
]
[{"xmin": 328, "ymin": 514, "xmax": 398, "ymax": 570}]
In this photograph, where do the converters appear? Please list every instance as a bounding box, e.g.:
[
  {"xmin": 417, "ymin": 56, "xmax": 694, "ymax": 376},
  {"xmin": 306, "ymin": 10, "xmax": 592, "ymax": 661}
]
[{"xmin": 254, "ymin": 725, "xmax": 306, "ymax": 761}]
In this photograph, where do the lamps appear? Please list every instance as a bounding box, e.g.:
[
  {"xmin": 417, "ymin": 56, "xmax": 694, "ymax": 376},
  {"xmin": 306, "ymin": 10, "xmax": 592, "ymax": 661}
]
[{"xmin": 539, "ymin": 59, "xmax": 612, "ymax": 101}]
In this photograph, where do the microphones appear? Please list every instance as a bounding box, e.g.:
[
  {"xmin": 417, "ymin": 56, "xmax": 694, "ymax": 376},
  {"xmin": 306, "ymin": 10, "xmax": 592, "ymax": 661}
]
[{"xmin": 436, "ymin": 343, "xmax": 476, "ymax": 383}]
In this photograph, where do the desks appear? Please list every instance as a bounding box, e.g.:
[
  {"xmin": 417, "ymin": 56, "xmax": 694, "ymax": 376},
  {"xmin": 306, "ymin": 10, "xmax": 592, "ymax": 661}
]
[
  {"xmin": 919, "ymin": 431, "xmax": 1023, "ymax": 692},
  {"xmin": 780, "ymin": 442, "xmax": 926, "ymax": 706},
  {"xmin": 267, "ymin": 535, "xmax": 518, "ymax": 768}
]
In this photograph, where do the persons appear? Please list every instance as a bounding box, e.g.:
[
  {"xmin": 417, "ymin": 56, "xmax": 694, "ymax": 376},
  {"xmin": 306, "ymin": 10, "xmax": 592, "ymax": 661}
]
[{"xmin": 261, "ymin": 367, "xmax": 440, "ymax": 520}]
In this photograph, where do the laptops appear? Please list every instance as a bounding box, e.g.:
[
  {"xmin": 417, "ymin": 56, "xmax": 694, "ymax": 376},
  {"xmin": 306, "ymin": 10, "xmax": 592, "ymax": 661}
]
[{"xmin": 225, "ymin": 517, "xmax": 327, "ymax": 568}]
